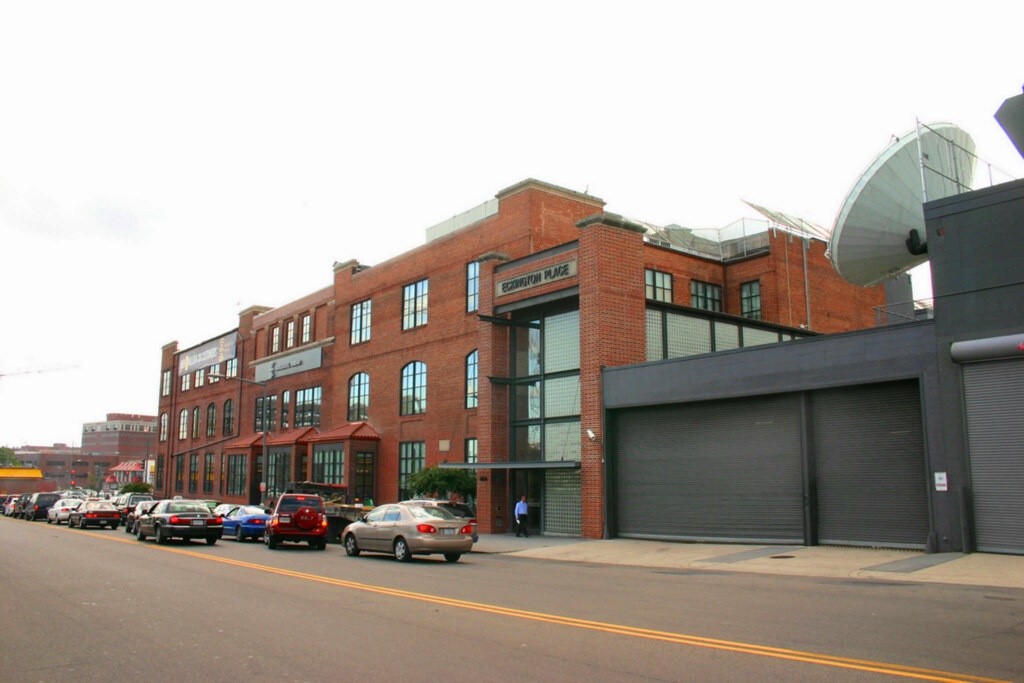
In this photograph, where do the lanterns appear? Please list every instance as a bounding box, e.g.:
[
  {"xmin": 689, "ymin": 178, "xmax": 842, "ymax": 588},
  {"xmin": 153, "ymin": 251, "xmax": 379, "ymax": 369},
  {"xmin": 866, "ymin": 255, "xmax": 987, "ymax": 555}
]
[
  {"xmin": 71, "ymin": 481, "xmax": 76, "ymax": 485},
  {"xmin": 70, "ymin": 470, "xmax": 76, "ymax": 474}
]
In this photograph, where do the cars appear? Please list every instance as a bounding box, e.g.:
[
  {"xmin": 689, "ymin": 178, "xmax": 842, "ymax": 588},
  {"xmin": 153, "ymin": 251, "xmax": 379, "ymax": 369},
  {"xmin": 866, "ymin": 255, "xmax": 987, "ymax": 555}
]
[
  {"xmin": 68, "ymin": 497, "xmax": 122, "ymax": 530},
  {"xmin": 0, "ymin": 494, "xmax": 22, "ymax": 517},
  {"xmin": 137, "ymin": 499, "xmax": 224, "ymax": 546},
  {"xmin": 52, "ymin": 484, "xmax": 119, "ymax": 500},
  {"xmin": 48, "ymin": 499, "xmax": 83, "ymax": 525},
  {"xmin": 323, "ymin": 501, "xmax": 369, "ymax": 540},
  {"xmin": 24, "ymin": 492, "xmax": 61, "ymax": 522},
  {"xmin": 13, "ymin": 494, "xmax": 32, "ymax": 519},
  {"xmin": 125, "ymin": 501, "xmax": 160, "ymax": 532},
  {"xmin": 342, "ymin": 503, "xmax": 473, "ymax": 563},
  {"xmin": 221, "ymin": 506, "xmax": 274, "ymax": 543}
]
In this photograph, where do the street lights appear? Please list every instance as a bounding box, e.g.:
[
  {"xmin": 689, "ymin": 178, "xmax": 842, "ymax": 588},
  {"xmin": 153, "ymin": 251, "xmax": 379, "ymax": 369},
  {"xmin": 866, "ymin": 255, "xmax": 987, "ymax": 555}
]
[{"xmin": 207, "ymin": 373, "xmax": 268, "ymax": 504}]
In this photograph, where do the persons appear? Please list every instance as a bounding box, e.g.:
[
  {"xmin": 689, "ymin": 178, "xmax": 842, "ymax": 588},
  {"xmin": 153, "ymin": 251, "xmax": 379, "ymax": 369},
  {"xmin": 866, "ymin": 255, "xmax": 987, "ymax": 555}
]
[{"xmin": 514, "ymin": 495, "xmax": 529, "ymax": 537}]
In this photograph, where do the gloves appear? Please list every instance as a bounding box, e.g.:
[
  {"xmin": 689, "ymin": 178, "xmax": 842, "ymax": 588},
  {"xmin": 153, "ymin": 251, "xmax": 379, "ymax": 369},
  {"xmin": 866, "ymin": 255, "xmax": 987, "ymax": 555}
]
[{"xmin": 516, "ymin": 519, "xmax": 520, "ymax": 524}]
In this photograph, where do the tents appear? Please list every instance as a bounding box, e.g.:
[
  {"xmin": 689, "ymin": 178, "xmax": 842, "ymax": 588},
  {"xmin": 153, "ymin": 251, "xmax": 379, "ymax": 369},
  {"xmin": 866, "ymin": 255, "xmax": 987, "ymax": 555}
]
[{"xmin": 108, "ymin": 462, "xmax": 141, "ymax": 483}]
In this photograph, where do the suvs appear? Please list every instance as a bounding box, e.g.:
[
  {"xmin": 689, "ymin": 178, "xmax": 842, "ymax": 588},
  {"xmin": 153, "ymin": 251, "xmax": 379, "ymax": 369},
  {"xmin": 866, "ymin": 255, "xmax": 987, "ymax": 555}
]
[
  {"xmin": 113, "ymin": 493, "xmax": 155, "ymax": 526},
  {"xmin": 401, "ymin": 499, "xmax": 479, "ymax": 543},
  {"xmin": 263, "ymin": 491, "xmax": 327, "ymax": 550}
]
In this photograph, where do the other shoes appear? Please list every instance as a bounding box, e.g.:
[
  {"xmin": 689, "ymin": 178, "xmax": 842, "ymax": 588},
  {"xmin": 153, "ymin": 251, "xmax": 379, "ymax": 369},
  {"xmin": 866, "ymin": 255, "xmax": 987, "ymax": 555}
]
[
  {"xmin": 517, "ymin": 534, "xmax": 522, "ymax": 537},
  {"xmin": 524, "ymin": 534, "xmax": 530, "ymax": 538}
]
